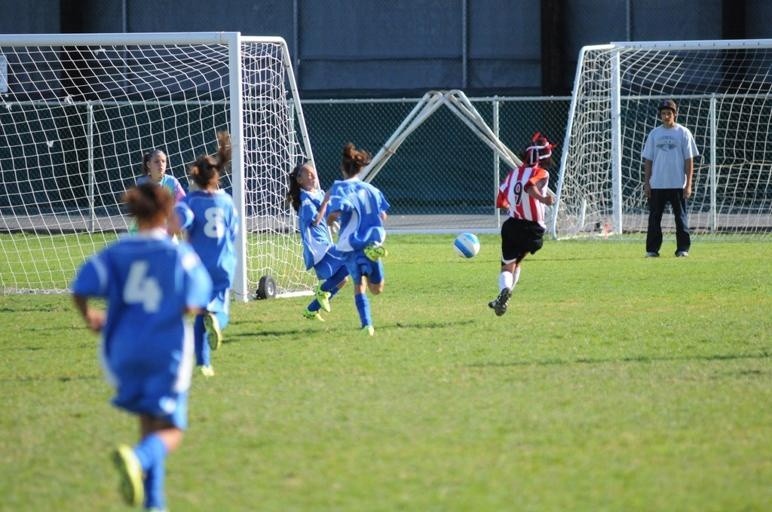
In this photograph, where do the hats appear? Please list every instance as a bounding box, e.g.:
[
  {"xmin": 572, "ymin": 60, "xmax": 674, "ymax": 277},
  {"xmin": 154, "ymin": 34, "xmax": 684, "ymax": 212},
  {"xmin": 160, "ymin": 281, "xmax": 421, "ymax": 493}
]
[{"xmin": 657, "ymin": 100, "xmax": 676, "ymax": 112}]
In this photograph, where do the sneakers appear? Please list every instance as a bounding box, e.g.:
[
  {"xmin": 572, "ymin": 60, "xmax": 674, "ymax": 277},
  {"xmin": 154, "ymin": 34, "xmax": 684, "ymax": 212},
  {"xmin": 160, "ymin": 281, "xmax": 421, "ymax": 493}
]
[
  {"xmin": 488, "ymin": 291, "xmax": 512, "ymax": 308},
  {"xmin": 495, "ymin": 287, "xmax": 509, "ymax": 316},
  {"xmin": 204, "ymin": 313, "xmax": 223, "ymax": 352},
  {"xmin": 195, "ymin": 364, "xmax": 215, "ymax": 376},
  {"xmin": 363, "ymin": 242, "xmax": 387, "ymax": 261},
  {"xmin": 312, "ymin": 280, "xmax": 331, "ymax": 312},
  {"xmin": 108, "ymin": 443, "xmax": 145, "ymax": 507},
  {"xmin": 301, "ymin": 307, "xmax": 325, "ymax": 323}
]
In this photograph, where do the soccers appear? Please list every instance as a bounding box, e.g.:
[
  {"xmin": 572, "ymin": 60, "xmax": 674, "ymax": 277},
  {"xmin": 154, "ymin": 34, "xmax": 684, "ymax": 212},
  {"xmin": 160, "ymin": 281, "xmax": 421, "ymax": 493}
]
[{"xmin": 453, "ymin": 232, "xmax": 481, "ymax": 259}]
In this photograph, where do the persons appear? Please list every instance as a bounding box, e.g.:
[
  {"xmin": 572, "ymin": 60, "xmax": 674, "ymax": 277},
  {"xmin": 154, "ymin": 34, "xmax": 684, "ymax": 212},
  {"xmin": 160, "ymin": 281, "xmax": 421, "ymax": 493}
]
[
  {"xmin": 488, "ymin": 133, "xmax": 556, "ymax": 316},
  {"xmin": 72, "ymin": 184, "xmax": 211, "ymax": 511},
  {"xmin": 175, "ymin": 134, "xmax": 238, "ymax": 376},
  {"xmin": 127, "ymin": 150, "xmax": 185, "ymax": 240},
  {"xmin": 327, "ymin": 142, "xmax": 390, "ymax": 334},
  {"xmin": 288, "ymin": 165, "xmax": 347, "ymax": 321},
  {"xmin": 641, "ymin": 101, "xmax": 699, "ymax": 258}
]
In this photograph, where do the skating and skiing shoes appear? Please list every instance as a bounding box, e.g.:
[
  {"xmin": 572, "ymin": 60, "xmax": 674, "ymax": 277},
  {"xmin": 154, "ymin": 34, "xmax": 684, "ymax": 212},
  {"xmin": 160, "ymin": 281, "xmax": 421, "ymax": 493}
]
[{"xmin": 360, "ymin": 326, "xmax": 375, "ymax": 336}]
